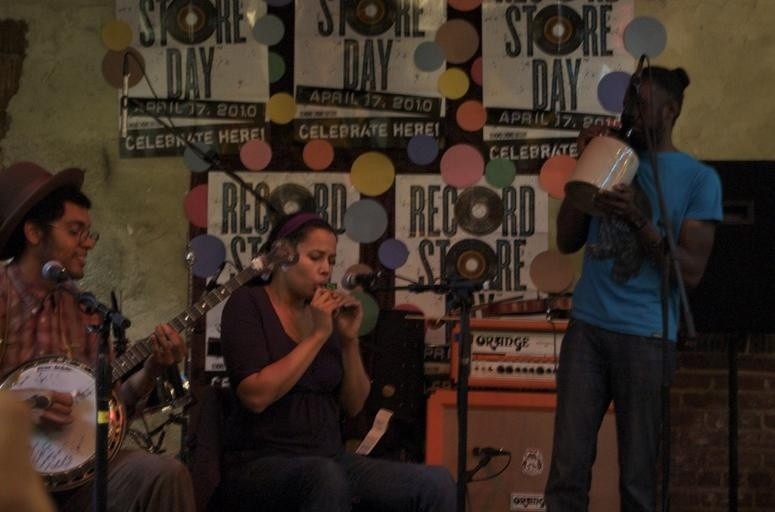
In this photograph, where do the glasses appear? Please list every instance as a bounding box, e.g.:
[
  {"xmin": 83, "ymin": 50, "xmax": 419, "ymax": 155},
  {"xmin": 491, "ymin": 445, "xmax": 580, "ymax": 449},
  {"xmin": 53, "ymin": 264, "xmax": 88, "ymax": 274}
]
[{"xmin": 46, "ymin": 223, "xmax": 100, "ymax": 244}]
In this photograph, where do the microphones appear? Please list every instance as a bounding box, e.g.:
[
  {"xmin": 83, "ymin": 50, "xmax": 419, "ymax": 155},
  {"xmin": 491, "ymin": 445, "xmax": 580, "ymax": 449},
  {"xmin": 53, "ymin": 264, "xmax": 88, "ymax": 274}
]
[
  {"xmin": 621, "ymin": 54, "xmax": 645, "ymax": 136},
  {"xmin": 42, "ymin": 260, "xmax": 64, "ymax": 289},
  {"xmin": 342, "ymin": 271, "xmax": 381, "ymax": 290},
  {"xmin": 121, "ymin": 56, "xmax": 129, "ymax": 138},
  {"xmin": 473, "ymin": 447, "xmax": 510, "ymax": 455}
]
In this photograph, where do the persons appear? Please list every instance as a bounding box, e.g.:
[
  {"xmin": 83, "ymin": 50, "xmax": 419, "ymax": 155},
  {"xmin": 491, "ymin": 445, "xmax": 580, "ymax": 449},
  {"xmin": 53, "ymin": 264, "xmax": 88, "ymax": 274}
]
[
  {"xmin": 217, "ymin": 210, "xmax": 460, "ymax": 512},
  {"xmin": 544, "ymin": 66, "xmax": 723, "ymax": 512},
  {"xmin": 0, "ymin": 160, "xmax": 199, "ymax": 511}
]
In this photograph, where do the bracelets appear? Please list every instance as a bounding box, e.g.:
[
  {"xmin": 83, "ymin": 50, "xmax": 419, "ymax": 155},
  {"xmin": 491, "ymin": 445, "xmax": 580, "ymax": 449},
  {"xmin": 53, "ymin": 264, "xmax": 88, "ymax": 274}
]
[{"xmin": 628, "ymin": 215, "xmax": 650, "ymax": 234}]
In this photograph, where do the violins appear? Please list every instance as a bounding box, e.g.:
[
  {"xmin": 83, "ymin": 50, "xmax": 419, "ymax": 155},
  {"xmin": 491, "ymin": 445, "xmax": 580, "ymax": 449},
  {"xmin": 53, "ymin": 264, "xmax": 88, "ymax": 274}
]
[{"xmin": 429, "ymin": 295, "xmax": 572, "ymax": 330}]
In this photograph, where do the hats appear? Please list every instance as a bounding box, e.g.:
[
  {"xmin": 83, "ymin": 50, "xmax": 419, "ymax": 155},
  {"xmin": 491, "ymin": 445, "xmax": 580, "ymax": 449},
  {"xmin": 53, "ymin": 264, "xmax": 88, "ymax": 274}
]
[
  {"xmin": 0, "ymin": 161, "xmax": 85, "ymax": 260},
  {"xmin": 631, "ymin": 67, "xmax": 692, "ymax": 100}
]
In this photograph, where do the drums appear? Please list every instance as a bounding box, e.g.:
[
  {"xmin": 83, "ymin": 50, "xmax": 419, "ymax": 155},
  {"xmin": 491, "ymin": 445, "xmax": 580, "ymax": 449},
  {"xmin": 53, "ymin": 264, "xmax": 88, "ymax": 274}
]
[{"xmin": 119, "ymin": 355, "xmax": 191, "ymax": 419}]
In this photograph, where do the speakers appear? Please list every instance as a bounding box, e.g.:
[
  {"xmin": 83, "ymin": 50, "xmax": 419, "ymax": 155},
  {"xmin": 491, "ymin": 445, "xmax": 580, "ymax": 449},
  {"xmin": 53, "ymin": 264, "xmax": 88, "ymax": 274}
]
[{"xmin": 425, "ymin": 388, "xmax": 623, "ymax": 512}]
